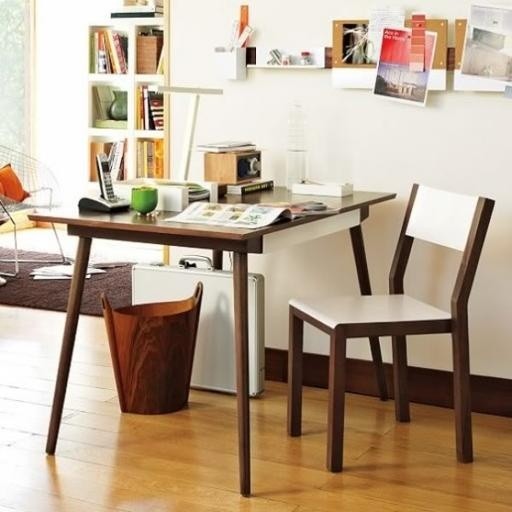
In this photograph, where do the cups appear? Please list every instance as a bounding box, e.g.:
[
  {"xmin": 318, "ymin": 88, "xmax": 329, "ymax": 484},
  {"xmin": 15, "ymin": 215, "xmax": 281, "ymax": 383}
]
[{"xmin": 130, "ymin": 187, "xmax": 159, "ymax": 214}]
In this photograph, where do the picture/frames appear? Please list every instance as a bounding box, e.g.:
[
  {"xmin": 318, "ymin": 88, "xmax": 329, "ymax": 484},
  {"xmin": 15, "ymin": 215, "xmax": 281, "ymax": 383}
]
[{"xmin": 332, "ymin": 21, "xmax": 376, "ymax": 67}]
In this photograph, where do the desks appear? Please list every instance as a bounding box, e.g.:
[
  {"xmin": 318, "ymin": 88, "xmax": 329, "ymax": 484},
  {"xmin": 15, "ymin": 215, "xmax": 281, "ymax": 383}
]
[{"xmin": 27, "ymin": 191, "xmax": 397, "ymax": 497}]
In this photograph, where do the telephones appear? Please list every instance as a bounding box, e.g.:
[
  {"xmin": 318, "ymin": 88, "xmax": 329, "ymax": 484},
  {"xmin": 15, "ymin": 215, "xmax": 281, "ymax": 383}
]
[{"xmin": 78, "ymin": 154, "xmax": 129, "ymax": 214}]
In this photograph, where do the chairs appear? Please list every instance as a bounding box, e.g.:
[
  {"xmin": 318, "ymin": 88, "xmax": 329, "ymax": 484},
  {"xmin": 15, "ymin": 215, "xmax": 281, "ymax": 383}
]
[
  {"xmin": 0, "ymin": 144, "xmax": 64, "ymax": 277},
  {"xmin": 287, "ymin": 183, "xmax": 495, "ymax": 474}
]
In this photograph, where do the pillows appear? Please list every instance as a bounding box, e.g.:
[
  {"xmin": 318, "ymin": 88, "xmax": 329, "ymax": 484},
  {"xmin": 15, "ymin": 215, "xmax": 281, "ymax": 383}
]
[{"xmin": 0, "ymin": 163, "xmax": 31, "ymax": 205}]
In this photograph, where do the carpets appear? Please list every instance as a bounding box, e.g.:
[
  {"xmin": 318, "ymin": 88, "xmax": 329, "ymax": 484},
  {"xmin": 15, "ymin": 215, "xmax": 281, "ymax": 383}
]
[{"xmin": 0, "ymin": 248, "xmax": 136, "ymax": 317}]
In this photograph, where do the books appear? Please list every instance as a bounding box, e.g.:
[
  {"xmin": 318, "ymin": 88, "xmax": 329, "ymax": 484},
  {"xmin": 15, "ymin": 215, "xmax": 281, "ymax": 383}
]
[
  {"xmin": 110, "ymin": 5, "xmax": 164, "ymax": 18},
  {"xmin": 227, "ymin": 178, "xmax": 275, "ymax": 195},
  {"xmin": 160, "ymin": 201, "xmax": 340, "ymax": 228},
  {"xmin": 90, "ymin": 28, "xmax": 165, "ymax": 180}
]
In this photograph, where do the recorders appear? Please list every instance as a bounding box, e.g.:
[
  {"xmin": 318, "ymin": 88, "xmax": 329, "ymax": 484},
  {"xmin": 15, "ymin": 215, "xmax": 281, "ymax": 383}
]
[{"xmin": 203, "ymin": 151, "xmax": 263, "ymax": 183}]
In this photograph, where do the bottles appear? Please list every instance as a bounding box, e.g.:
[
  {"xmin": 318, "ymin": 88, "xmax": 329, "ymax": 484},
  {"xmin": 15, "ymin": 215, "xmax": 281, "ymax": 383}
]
[
  {"xmin": 300, "ymin": 52, "xmax": 312, "ymax": 65},
  {"xmin": 282, "ymin": 55, "xmax": 290, "ymax": 66}
]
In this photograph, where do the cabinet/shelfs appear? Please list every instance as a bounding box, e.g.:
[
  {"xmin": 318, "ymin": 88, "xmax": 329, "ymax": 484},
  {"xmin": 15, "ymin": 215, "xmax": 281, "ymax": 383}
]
[{"xmin": 86, "ymin": 0, "xmax": 170, "ymax": 185}]
